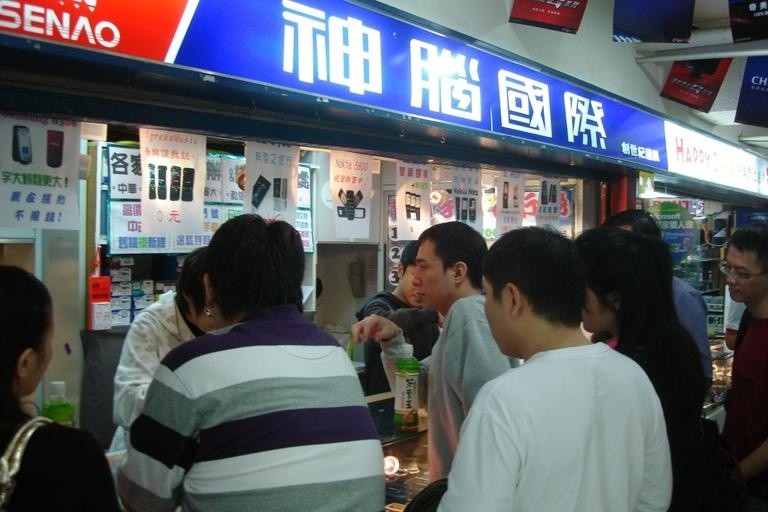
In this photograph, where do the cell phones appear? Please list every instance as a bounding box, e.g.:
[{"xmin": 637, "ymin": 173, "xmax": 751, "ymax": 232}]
[
  {"xmin": 405, "ymin": 192, "xmax": 420, "ymax": 220},
  {"xmin": 455, "ymin": 197, "xmax": 476, "ymax": 221},
  {"xmin": 148, "ymin": 163, "xmax": 195, "ymax": 201},
  {"xmin": 502, "ymin": 181, "xmax": 519, "ymax": 209},
  {"xmin": 541, "ymin": 181, "xmax": 556, "ymax": 205},
  {"xmin": 47, "ymin": 130, "xmax": 64, "ymax": 168},
  {"xmin": 12, "ymin": 126, "xmax": 32, "ymax": 165},
  {"xmin": 252, "ymin": 175, "xmax": 289, "ymax": 212},
  {"xmin": 337, "ymin": 188, "xmax": 366, "ymax": 221}
]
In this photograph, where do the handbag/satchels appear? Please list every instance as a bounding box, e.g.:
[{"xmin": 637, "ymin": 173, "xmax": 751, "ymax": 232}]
[{"xmin": 671, "ymin": 415, "xmax": 746, "ymax": 512}]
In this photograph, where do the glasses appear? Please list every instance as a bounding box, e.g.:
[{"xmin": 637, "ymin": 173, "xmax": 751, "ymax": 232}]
[{"xmin": 717, "ymin": 259, "xmax": 752, "ymax": 279}]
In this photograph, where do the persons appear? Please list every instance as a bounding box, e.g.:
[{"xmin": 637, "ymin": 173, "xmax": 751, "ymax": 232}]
[
  {"xmin": 350, "ymin": 210, "xmax": 713, "ymax": 512},
  {"xmin": 110, "ymin": 247, "xmax": 217, "ymax": 452},
  {"xmin": 0, "ymin": 262, "xmax": 122, "ymax": 511},
  {"xmin": 118, "ymin": 214, "xmax": 384, "ymax": 511},
  {"xmin": 724, "ymin": 299, "xmax": 746, "ymax": 350},
  {"xmin": 718, "ymin": 219, "xmax": 768, "ymax": 512}
]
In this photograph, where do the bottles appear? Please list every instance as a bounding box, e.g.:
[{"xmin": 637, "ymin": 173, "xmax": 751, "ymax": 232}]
[
  {"xmin": 392, "ymin": 343, "xmax": 420, "ymax": 427},
  {"xmin": 40, "ymin": 379, "xmax": 77, "ymax": 428}
]
